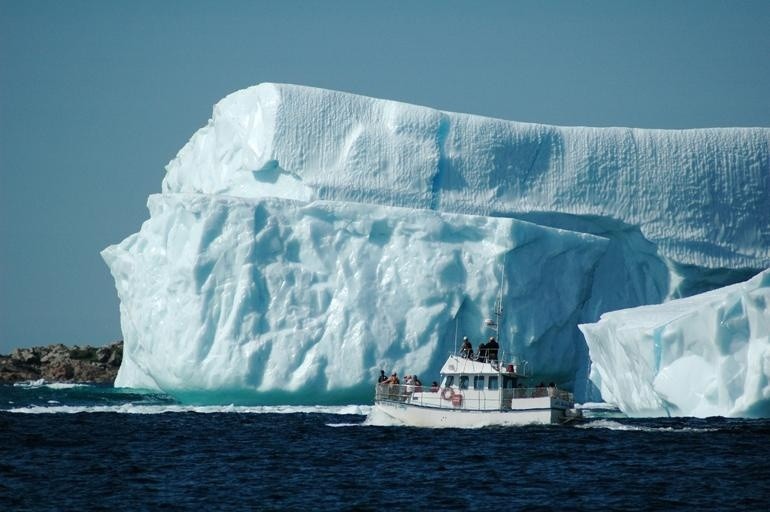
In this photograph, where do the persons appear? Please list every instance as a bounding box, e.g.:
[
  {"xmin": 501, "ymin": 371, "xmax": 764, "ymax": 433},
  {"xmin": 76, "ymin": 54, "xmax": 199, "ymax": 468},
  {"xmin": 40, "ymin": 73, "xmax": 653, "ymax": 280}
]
[{"xmin": 377, "ymin": 336, "xmax": 554, "ymax": 404}]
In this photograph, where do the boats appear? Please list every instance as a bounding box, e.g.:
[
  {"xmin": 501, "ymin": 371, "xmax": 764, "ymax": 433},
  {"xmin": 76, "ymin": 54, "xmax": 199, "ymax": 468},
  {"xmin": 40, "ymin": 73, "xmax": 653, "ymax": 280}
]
[{"xmin": 368, "ymin": 243, "xmax": 588, "ymax": 427}]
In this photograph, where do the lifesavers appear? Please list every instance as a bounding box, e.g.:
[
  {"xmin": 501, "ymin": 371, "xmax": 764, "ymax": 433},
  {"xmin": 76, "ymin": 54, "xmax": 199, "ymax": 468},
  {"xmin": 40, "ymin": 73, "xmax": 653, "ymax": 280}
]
[{"xmin": 441, "ymin": 387, "xmax": 454, "ymax": 400}]
